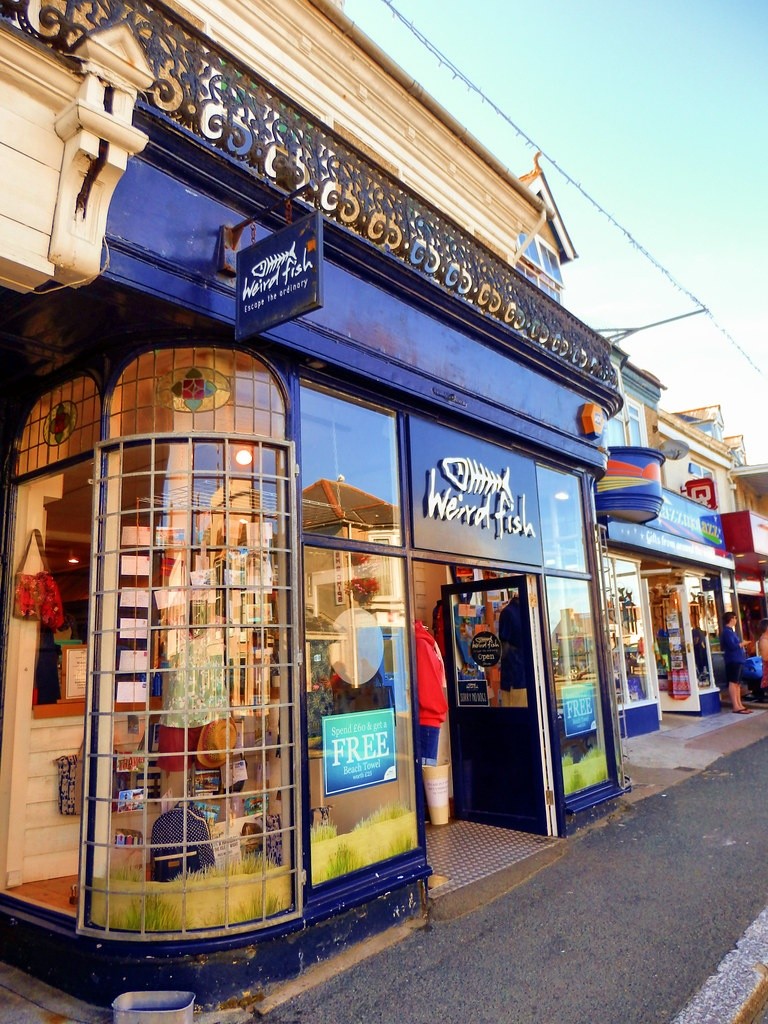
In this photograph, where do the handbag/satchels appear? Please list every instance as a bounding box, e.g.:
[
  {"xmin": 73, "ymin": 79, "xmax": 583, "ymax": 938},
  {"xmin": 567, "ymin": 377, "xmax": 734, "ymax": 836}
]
[
  {"xmin": 12, "ymin": 526, "xmax": 63, "ymax": 631},
  {"xmin": 52, "ymin": 737, "xmax": 84, "ymax": 816}
]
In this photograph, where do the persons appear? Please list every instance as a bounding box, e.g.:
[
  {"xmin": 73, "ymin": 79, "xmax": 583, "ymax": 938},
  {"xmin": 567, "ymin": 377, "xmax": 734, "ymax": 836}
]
[
  {"xmin": 721, "ymin": 612, "xmax": 768, "ymax": 714},
  {"xmin": 498, "ymin": 594, "xmax": 530, "ymax": 708},
  {"xmin": 414, "ymin": 620, "xmax": 448, "ymax": 823},
  {"xmin": 155, "ymin": 586, "xmax": 230, "ymax": 773}
]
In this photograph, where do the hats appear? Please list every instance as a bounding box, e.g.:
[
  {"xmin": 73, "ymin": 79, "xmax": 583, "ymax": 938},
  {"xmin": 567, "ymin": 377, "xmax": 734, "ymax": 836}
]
[{"xmin": 197, "ymin": 714, "xmax": 237, "ymax": 766}]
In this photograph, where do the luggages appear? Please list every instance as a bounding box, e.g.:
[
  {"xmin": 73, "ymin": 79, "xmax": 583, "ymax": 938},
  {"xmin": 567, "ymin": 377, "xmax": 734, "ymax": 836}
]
[{"xmin": 150, "ymin": 799, "xmax": 216, "ymax": 882}]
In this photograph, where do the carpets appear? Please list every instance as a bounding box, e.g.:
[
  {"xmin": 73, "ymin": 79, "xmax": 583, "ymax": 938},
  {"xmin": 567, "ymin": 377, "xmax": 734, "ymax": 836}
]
[{"xmin": 424, "ymin": 818, "xmax": 563, "ymax": 898}]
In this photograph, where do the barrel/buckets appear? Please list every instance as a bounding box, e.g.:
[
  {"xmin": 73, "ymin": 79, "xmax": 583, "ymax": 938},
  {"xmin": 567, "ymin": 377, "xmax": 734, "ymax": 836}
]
[
  {"xmin": 111, "ymin": 991, "xmax": 196, "ymax": 1024},
  {"xmin": 422, "ymin": 762, "xmax": 451, "ymax": 826}
]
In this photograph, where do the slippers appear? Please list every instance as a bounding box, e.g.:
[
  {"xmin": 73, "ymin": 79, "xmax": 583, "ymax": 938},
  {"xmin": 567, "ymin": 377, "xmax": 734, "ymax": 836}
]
[{"xmin": 732, "ymin": 708, "xmax": 753, "ymax": 714}]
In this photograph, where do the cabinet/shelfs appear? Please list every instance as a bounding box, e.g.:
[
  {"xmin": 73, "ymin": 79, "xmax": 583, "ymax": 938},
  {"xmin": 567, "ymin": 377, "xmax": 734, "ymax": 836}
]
[
  {"xmin": 607, "ymin": 596, "xmax": 700, "ymax": 646},
  {"xmin": 133, "ymin": 488, "xmax": 359, "ymax": 835}
]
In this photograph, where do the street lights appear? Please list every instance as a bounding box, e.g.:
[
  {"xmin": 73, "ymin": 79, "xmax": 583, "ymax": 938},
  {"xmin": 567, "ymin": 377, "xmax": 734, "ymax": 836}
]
[{"xmin": 549, "ymin": 490, "xmax": 571, "ymax": 683}]
